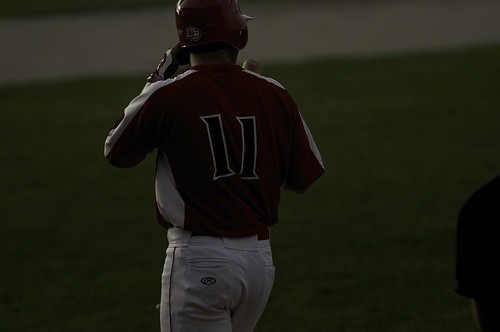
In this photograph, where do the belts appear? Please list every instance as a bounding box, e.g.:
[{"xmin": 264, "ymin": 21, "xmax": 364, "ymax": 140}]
[{"xmin": 192, "ymin": 229, "xmax": 269, "ymax": 241}]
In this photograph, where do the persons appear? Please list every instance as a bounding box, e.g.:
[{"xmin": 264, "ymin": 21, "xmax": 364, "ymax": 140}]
[
  {"xmin": 456, "ymin": 177, "xmax": 500, "ymax": 332},
  {"xmin": 105, "ymin": 0, "xmax": 324, "ymax": 332}
]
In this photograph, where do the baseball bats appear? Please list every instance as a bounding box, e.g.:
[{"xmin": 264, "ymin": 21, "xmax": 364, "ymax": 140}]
[{"xmin": 242, "ymin": 57, "xmax": 265, "ymax": 75}]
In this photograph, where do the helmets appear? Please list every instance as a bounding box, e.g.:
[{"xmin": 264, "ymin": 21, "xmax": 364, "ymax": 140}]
[{"xmin": 176, "ymin": 1, "xmax": 254, "ymax": 51}]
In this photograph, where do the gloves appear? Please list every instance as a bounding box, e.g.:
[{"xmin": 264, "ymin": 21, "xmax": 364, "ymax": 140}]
[{"xmin": 146, "ymin": 43, "xmax": 189, "ymax": 85}]
